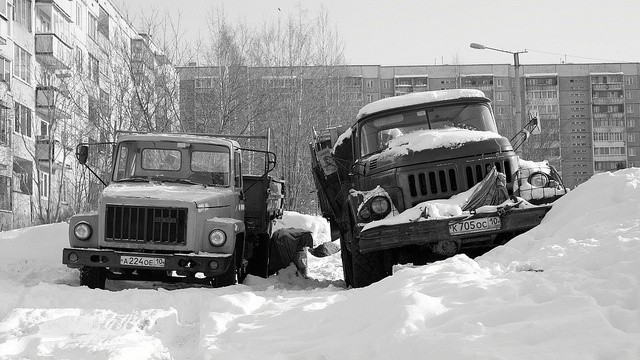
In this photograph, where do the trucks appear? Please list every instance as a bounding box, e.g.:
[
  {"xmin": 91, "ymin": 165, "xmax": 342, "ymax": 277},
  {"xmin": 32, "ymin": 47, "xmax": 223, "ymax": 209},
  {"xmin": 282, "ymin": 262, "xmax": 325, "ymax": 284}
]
[
  {"xmin": 63, "ymin": 127, "xmax": 285, "ymax": 289},
  {"xmin": 308, "ymin": 96, "xmax": 567, "ymax": 288}
]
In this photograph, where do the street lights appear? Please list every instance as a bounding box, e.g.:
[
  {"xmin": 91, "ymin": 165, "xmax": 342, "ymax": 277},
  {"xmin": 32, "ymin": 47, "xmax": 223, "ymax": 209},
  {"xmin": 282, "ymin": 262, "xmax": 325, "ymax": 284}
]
[{"xmin": 470, "ymin": 43, "xmax": 529, "ymax": 159}]
